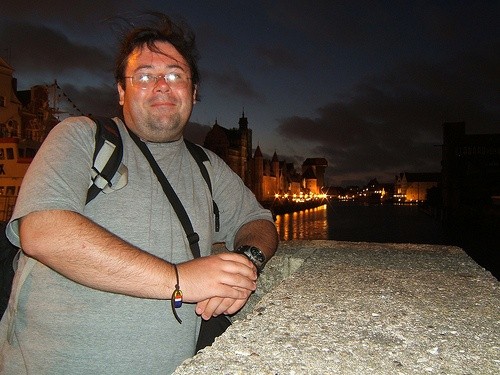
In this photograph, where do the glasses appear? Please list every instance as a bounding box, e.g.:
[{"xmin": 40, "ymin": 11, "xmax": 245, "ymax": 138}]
[{"xmin": 119, "ymin": 70, "xmax": 193, "ymax": 90}]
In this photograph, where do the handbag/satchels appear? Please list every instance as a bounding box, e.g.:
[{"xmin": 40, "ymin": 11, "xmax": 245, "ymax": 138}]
[{"xmin": 195, "ymin": 312, "xmax": 231, "ymax": 353}]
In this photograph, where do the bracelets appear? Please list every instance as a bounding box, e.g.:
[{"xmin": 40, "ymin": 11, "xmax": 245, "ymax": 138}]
[{"xmin": 171, "ymin": 262, "xmax": 184, "ymax": 324}]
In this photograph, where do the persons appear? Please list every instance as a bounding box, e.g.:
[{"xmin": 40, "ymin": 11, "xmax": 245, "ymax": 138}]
[{"xmin": 1, "ymin": 20, "xmax": 277, "ymax": 375}]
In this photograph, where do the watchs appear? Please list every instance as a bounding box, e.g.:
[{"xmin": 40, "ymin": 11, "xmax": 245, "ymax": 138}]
[{"xmin": 234, "ymin": 244, "xmax": 266, "ymax": 274}]
